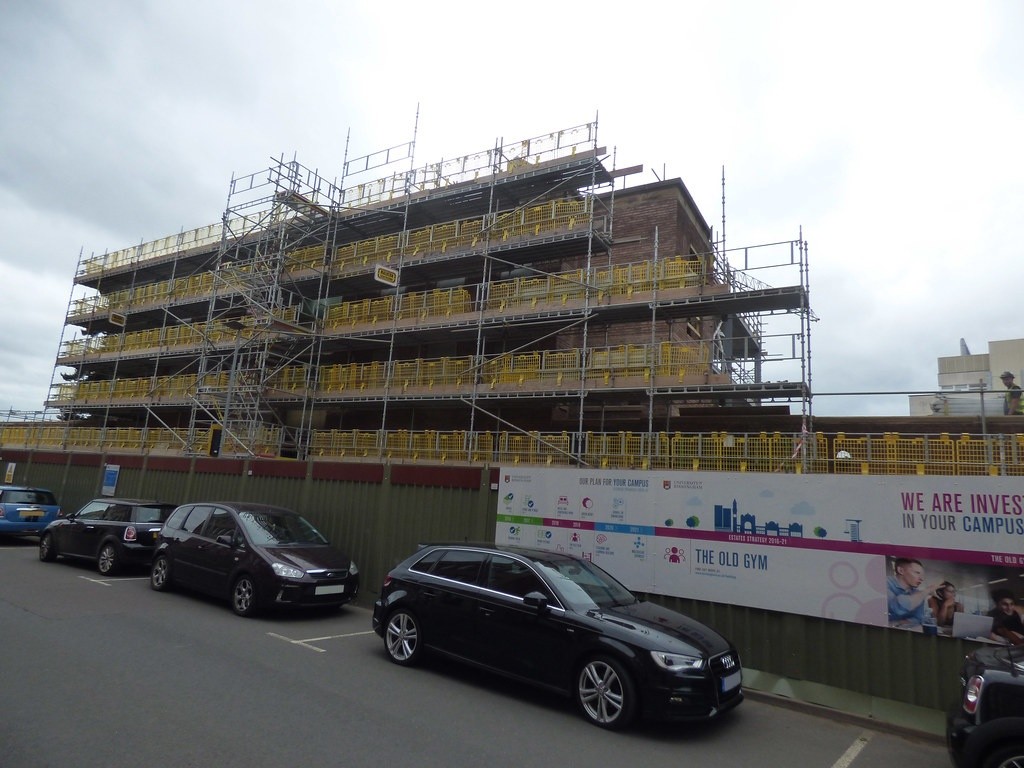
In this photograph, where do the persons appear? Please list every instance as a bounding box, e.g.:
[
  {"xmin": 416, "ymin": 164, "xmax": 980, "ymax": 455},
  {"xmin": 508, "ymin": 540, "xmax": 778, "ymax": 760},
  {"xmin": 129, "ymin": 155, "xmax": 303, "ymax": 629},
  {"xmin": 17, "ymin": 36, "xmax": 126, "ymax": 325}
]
[
  {"xmin": 984, "ymin": 590, "xmax": 1024, "ymax": 646},
  {"xmin": 1000, "ymin": 371, "xmax": 1024, "ymax": 417},
  {"xmin": 887, "ymin": 558, "xmax": 964, "ymax": 628}
]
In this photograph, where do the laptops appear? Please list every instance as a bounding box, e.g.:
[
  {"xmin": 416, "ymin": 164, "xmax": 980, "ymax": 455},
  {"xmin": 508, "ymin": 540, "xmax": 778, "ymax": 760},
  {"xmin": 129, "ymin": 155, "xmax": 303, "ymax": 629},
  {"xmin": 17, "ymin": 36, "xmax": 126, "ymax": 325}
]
[{"xmin": 943, "ymin": 612, "xmax": 994, "ymax": 638}]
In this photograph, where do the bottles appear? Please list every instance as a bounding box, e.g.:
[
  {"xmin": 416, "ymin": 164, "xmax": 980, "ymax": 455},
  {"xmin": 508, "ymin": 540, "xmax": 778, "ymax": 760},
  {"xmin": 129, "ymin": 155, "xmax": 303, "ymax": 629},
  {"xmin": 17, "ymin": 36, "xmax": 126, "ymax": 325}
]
[{"xmin": 922, "ymin": 607, "xmax": 937, "ymax": 635}]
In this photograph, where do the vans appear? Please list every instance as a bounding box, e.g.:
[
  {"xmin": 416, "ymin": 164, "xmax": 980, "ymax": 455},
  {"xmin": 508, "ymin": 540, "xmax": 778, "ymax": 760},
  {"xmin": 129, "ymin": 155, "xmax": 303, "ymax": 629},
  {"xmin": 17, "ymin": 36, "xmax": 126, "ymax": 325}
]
[{"xmin": 150, "ymin": 501, "xmax": 360, "ymax": 618}]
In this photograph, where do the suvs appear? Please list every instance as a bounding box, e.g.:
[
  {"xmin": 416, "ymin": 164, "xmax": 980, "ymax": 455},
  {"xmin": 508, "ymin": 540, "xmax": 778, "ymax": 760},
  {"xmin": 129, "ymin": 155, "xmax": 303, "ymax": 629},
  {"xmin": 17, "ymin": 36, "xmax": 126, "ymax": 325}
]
[{"xmin": 372, "ymin": 544, "xmax": 746, "ymax": 732}]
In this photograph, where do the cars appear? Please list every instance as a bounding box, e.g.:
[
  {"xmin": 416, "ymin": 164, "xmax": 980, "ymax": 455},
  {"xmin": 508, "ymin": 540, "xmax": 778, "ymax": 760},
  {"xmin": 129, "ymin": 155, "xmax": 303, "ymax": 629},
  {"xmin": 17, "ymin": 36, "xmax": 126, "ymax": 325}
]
[
  {"xmin": 39, "ymin": 497, "xmax": 181, "ymax": 578},
  {"xmin": 0, "ymin": 485, "xmax": 63, "ymax": 545},
  {"xmin": 946, "ymin": 643, "xmax": 1023, "ymax": 768}
]
